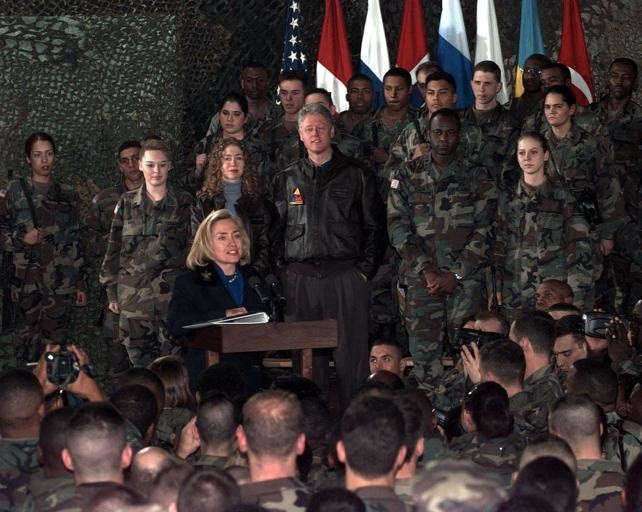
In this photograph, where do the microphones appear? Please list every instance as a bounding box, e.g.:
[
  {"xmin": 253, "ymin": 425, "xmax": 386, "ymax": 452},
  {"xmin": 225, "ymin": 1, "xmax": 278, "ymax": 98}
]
[
  {"xmin": 248, "ymin": 275, "xmax": 270, "ymax": 304},
  {"xmin": 265, "ymin": 274, "xmax": 287, "ymax": 305}
]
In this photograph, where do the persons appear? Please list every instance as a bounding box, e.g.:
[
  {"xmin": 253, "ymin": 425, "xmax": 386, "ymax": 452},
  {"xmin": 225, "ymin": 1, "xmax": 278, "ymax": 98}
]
[{"xmin": 1, "ymin": 54, "xmax": 641, "ymax": 512}]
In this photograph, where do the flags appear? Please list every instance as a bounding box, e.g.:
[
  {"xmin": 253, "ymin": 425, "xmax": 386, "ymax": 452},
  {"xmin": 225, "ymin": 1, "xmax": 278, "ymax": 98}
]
[
  {"xmin": 274, "ymin": 0, "xmax": 312, "ymax": 114},
  {"xmin": 316, "ymin": 0, "xmax": 354, "ymax": 113},
  {"xmin": 558, "ymin": 0, "xmax": 597, "ymax": 106},
  {"xmin": 357, "ymin": 0, "xmax": 391, "ymax": 115},
  {"xmin": 436, "ymin": 0, "xmax": 477, "ymax": 112},
  {"xmin": 396, "ymin": 0, "xmax": 429, "ymax": 109},
  {"xmin": 515, "ymin": 0, "xmax": 546, "ymax": 100},
  {"xmin": 473, "ymin": 0, "xmax": 511, "ymax": 105}
]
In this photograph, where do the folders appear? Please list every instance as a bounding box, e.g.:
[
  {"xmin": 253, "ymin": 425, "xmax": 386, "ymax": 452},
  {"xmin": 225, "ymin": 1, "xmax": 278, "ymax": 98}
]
[{"xmin": 183, "ymin": 311, "xmax": 270, "ymax": 329}]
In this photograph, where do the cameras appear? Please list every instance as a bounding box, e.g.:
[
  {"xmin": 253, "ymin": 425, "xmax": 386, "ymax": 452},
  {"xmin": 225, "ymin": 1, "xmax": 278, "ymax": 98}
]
[
  {"xmin": 451, "ymin": 327, "xmax": 507, "ymax": 354},
  {"xmin": 583, "ymin": 312, "xmax": 639, "ymax": 340},
  {"xmin": 45, "ymin": 350, "xmax": 80, "ymax": 385},
  {"xmin": 432, "ymin": 404, "xmax": 462, "ymax": 442}
]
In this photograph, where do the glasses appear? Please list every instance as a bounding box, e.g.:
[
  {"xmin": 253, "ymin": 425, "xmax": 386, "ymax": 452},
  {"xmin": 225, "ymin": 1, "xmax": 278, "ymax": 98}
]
[{"xmin": 521, "ymin": 68, "xmax": 541, "ymax": 76}]
